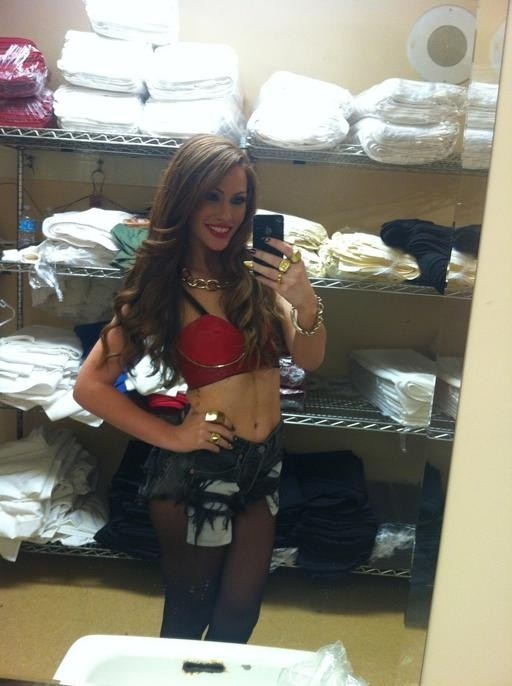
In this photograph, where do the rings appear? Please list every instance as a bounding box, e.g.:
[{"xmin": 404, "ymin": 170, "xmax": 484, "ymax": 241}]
[
  {"xmin": 208, "ymin": 432, "xmax": 221, "ymax": 445},
  {"xmin": 288, "ymin": 250, "xmax": 302, "ymax": 263},
  {"xmin": 278, "ymin": 258, "xmax": 292, "ymax": 273},
  {"xmin": 276, "ymin": 272, "xmax": 283, "ymax": 284},
  {"xmin": 204, "ymin": 410, "xmax": 225, "ymax": 424}
]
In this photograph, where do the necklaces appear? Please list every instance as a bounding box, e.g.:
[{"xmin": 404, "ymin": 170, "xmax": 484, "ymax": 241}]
[{"xmin": 179, "ymin": 264, "xmax": 229, "ymax": 290}]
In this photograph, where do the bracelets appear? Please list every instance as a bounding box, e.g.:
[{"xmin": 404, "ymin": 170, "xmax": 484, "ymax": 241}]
[{"xmin": 290, "ymin": 294, "xmax": 325, "ymax": 338}]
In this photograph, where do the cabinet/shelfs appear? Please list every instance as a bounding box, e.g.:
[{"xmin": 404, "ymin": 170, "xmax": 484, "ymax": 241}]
[{"xmin": 0, "ymin": 120, "xmax": 489, "ymax": 629}]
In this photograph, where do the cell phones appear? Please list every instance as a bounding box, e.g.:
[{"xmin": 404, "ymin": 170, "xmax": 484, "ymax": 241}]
[{"xmin": 253, "ymin": 215, "xmax": 284, "ymax": 274}]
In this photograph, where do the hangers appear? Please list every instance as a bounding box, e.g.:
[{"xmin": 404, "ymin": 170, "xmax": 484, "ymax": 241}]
[
  {"xmin": 50, "ymin": 168, "xmax": 132, "ymax": 214},
  {"xmin": 0, "ymin": 299, "xmax": 16, "ymax": 327}
]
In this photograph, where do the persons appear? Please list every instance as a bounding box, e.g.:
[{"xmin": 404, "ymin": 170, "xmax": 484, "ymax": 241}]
[{"xmin": 73, "ymin": 130, "xmax": 327, "ymax": 645}]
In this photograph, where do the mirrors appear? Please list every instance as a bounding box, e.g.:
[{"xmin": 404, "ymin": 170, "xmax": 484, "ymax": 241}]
[{"xmin": 0, "ymin": 0, "xmax": 508, "ymax": 686}]
